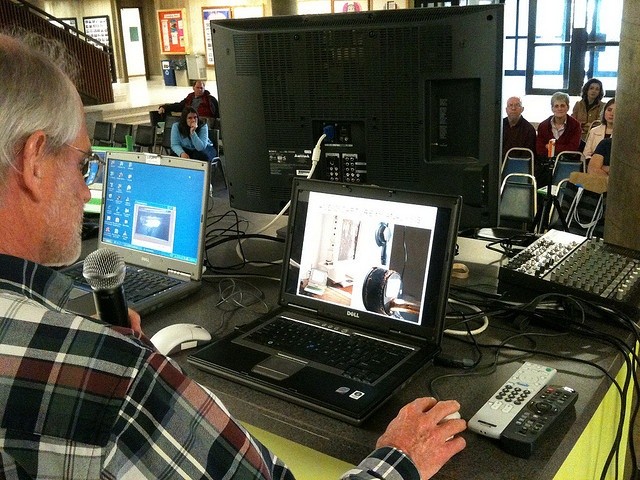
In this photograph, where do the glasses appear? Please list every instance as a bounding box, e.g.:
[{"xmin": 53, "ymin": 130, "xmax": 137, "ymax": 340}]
[{"xmin": 61, "ymin": 140, "xmax": 105, "ymax": 185}]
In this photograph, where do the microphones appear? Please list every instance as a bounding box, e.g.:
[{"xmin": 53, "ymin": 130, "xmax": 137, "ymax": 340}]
[{"xmin": 80, "ymin": 246, "xmax": 131, "ymax": 331}]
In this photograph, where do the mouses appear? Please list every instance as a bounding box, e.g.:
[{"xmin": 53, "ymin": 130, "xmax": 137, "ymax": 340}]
[{"xmin": 149, "ymin": 323, "xmax": 213, "ymax": 355}]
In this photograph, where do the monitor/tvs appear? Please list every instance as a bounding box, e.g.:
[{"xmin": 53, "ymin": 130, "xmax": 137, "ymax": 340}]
[{"xmin": 209, "ymin": 2, "xmax": 505, "ymax": 241}]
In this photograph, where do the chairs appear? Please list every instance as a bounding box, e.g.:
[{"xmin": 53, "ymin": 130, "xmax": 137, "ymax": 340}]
[
  {"xmin": 134, "ymin": 124, "xmax": 157, "ymax": 157},
  {"xmin": 500, "ymin": 147, "xmax": 537, "ymax": 194},
  {"xmin": 112, "ymin": 122, "xmax": 132, "ymax": 153},
  {"xmin": 550, "ymin": 150, "xmax": 588, "ymax": 187},
  {"xmin": 545, "ymin": 179, "xmax": 605, "ymax": 253},
  {"xmin": 476, "ymin": 174, "xmax": 536, "ymax": 244},
  {"xmin": 147, "ymin": 109, "xmax": 173, "ymax": 152},
  {"xmin": 177, "ymin": 126, "xmax": 227, "ymax": 192},
  {"xmin": 90, "ymin": 122, "xmax": 112, "ymax": 156}
]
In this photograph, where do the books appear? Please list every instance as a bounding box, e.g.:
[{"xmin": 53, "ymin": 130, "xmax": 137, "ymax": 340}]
[{"xmin": 537, "ymin": 185, "xmax": 565, "ymax": 196}]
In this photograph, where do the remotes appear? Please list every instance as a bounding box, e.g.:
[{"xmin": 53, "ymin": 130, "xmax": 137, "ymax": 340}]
[
  {"xmin": 500, "ymin": 382, "xmax": 580, "ymax": 460},
  {"xmin": 467, "ymin": 360, "xmax": 556, "ymax": 438}
]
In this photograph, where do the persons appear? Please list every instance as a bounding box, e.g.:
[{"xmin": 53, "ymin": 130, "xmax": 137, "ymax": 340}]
[
  {"xmin": 587, "ymin": 138, "xmax": 613, "ymax": 178},
  {"xmin": 537, "ymin": 92, "xmax": 582, "ymax": 158},
  {"xmin": 581, "ymin": 99, "xmax": 616, "ymax": 165},
  {"xmin": 503, "ymin": 97, "xmax": 537, "ymax": 159},
  {"xmin": 158, "ymin": 80, "xmax": 220, "ymax": 117},
  {"xmin": 171, "ymin": 106, "xmax": 218, "ymax": 162},
  {"xmin": 0, "ymin": 32, "xmax": 468, "ymax": 480},
  {"xmin": 571, "ymin": 79, "xmax": 608, "ymax": 143}
]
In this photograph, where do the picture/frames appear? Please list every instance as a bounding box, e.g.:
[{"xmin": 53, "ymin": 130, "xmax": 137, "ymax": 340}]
[
  {"xmin": 201, "ymin": 8, "xmax": 233, "ymax": 67},
  {"xmin": 331, "ymin": 0, "xmax": 374, "ymax": 14}
]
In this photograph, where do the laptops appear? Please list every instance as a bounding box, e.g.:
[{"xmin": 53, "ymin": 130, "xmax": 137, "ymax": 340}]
[
  {"xmin": 55, "ymin": 151, "xmax": 211, "ymax": 325},
  {"xmin": 185, "ymin": 176, "xmax": 463, "ymax": 429}
]
